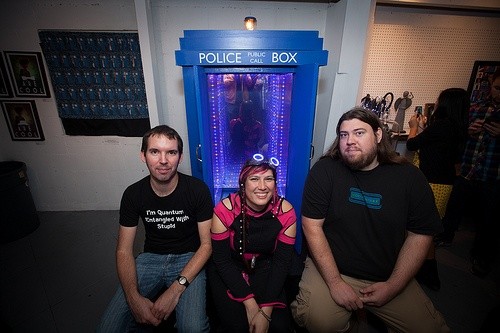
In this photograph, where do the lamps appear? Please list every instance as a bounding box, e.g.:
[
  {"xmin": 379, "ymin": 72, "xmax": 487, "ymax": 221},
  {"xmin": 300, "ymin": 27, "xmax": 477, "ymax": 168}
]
[{"xmin": 244, "ymin": 16, "xmax": 258, "ymax": 31}]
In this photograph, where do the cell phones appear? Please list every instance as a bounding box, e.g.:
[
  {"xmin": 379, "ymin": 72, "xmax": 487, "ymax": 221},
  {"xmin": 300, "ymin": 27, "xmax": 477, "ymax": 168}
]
[{"xmin": 415, "ymin": 106, "xmax": 422, "ymax": 118}]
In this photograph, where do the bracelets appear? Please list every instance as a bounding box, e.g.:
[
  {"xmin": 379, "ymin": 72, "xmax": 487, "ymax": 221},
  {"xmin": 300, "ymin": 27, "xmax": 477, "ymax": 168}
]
[{"xmin": 258, "ymin": 308, "xmax": 272, "ymax": 322}]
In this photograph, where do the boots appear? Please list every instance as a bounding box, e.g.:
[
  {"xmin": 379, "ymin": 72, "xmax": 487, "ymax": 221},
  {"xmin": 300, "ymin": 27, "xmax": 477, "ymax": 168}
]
[{"xmin": 417, "ymin": 257, "xmax": 443, "ymax": 292}]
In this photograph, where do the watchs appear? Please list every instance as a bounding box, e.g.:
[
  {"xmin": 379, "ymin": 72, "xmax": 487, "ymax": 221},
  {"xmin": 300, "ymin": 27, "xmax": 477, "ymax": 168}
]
[{"xmin": 177, "ymin": 276, "xmax": 189, "ymax": 287}]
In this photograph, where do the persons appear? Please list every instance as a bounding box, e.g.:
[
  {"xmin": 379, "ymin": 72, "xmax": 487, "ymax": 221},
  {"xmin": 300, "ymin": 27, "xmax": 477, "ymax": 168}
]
[
  {"xmin": 406, "ymin": 88, "xmax": 471, "ymax": 229},
  {"xmin": 96, "ymin": 125, "xmax": 214, "ymax": 332},
  {"xmin": 209, "ymin": 157, "xmax": 300, "ymax": 333},
  {"xmin": 459, "ymin": 69, "xmax": 500, "ymax": 265},
  {"xmin": 289, "ymin": 108, "xmax": 455, "ymax": 333}
]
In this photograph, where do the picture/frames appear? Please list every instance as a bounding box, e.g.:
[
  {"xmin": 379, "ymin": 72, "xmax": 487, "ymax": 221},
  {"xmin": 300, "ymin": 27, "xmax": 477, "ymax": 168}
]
[
  {"xmin": 0, "ymin": 52, "xmax": 14, "ymax": 98},
  {"xmin": 4, "ymin": 51, "xmax": 51, "ymax": 98},
  {"xmin": 0, "ymin": 100, "xmax": 45, "ymax": 142}
]
